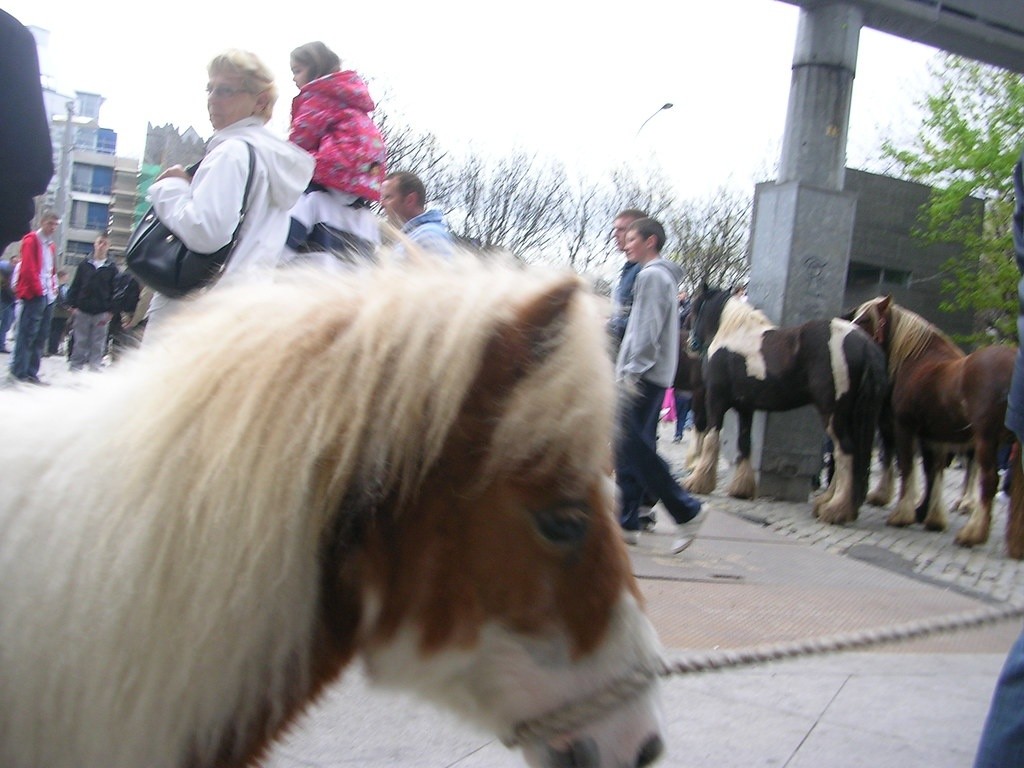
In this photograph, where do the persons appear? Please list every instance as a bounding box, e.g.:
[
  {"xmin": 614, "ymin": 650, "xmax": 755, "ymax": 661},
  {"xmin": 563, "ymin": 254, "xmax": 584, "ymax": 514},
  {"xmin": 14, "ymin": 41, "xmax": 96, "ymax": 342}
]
[
  {"xmin": 67, "ymin": 233, "xmax": 155, "ymax": 373},
  {"xmin": 287, "ymin": 41, "xmax": 387, "ymax": 202},
  {"xmin": 9, "ymin": 208, "xmax": 60, "ymax": 384},
  {"xmin": 380, "ymin": 171, "xmax": 454, "ymax": 259},
  {"xmin": 276, "ymin": 182, "xmax": 380, "ymax": 267},
  {"xmin": 141, "ymin": 50, "xmax": 316, "ymax": 344},
  {"xmin": 603, "ymin": 210, "xmax": 709, "ymax": 554},
  {"xmin": 971, "ymin": 154, "xmax": 1024, "ymax": 768},
  {"xmin": 0, "ymin": 255, "xmax": 17, "ymax": 354},
  {"xmin": 0, "ymin": 8, "xmax": 54, "ymax": 256},
  {"xmin": 49, "ymin": 270, "xmax": 69, "ymax": 356}
]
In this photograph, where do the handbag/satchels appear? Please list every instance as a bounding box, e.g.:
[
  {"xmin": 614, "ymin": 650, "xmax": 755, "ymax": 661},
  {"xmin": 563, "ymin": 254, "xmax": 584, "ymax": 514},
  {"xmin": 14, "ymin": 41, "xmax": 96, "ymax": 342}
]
[{"xmin": 125, "ymin": 141, "xmax": 256, "ymax": 300}]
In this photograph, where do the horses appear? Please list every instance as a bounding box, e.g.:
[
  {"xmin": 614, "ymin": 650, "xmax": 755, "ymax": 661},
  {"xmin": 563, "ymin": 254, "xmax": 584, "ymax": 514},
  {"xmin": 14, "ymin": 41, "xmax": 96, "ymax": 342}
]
[
  {"xmin": 0, "ymin": 231, "xmax": 668, "ymax": 768},
  {"xmin": 672, "ymin": 263, "xmax": 1023, "ymax": 563}
]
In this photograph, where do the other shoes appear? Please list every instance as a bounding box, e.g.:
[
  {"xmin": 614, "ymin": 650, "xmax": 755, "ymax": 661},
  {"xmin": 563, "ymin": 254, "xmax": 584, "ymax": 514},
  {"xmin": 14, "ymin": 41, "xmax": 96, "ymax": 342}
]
[
  {"xmin": 671, "ymin": 502, "xmax": 709, "ymax": 555},
  {"xmin": 8, "ymin": 372, "xmax": 47, "ymax": 387},
  {"xmin": 617, "ymin": 527, "xmax": 640, "ymax": 545},
  {"xmin": 638, "ymin": 511, "xmax": 657, "ymax": 532}
]
